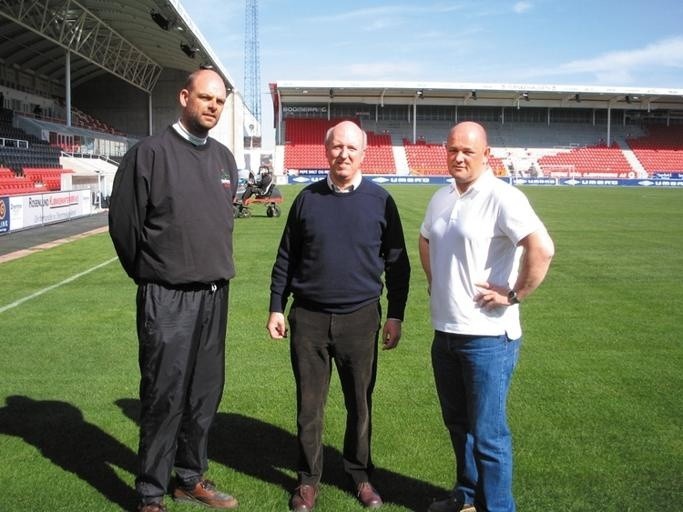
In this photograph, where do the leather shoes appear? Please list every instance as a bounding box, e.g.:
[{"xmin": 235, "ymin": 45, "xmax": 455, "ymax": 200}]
[{"xmin": 291, "ymin": 481, "xmax": 383, "ymax": 511}]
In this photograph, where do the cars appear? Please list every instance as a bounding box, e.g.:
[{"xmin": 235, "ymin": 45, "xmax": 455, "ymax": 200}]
[{"xmin": 235, "ymin": 167, "xmax": 255, "ymax": 197}]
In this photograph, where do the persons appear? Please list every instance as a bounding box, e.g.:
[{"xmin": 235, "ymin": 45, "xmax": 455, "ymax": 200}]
[
  {"xmin": 268, "ymin": 119, "xmax": 411, "ymax": 512},
  {"xmin": 107, "ymin": 69, "xmax": 239, "ymax": 511},
  {"xmin": 238, "ymin": 166, "xmax": 272, "ymax": 204},
  {"xmin": 419, "ymin": 121, "xmax": 554, "ymax": 512}
]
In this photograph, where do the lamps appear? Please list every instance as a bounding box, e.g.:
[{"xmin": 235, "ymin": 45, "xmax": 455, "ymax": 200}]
[
  {"xmin": 181, "ymin": 44, "xmax": 195, "ymax": 58},
  {"xmin": 151, "ymin": 13, "xmax": 169, "ymax": 30}
]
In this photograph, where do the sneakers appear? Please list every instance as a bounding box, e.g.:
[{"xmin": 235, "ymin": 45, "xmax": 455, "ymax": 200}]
[
  {"xmin": 427, "ymin": 495, "xmax": 483, "ymax": 512},
  {"xmin": 137, "ymin": 478, "xmax": 238, "ymax": 511}
]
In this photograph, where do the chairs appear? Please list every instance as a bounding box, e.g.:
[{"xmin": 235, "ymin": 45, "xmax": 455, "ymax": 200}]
[
  {"xmin": 256, "ymin": 184, "xmax": 275, "ymax": 198},
  {"xmin": 0, "ymin": 92, "xmax": 128, "ymax": 195},
  {"xmin": 537, "ymin": 124, "xmax": 683, "ymax": 178},
  {"xmin": 283, "ymin": 117, "xmax": 505, "ymax": 174}
]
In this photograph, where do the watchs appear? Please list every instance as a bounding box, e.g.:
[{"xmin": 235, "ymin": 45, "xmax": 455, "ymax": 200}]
[{"xmin": 508, "ymin": 290, "xmax": 520, "ymax": 304}]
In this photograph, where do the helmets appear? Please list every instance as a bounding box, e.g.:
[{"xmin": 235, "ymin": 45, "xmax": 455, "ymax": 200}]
[{"xmin": 259, "ymin": 166, "xmax": 269, "ymax": 174}]
